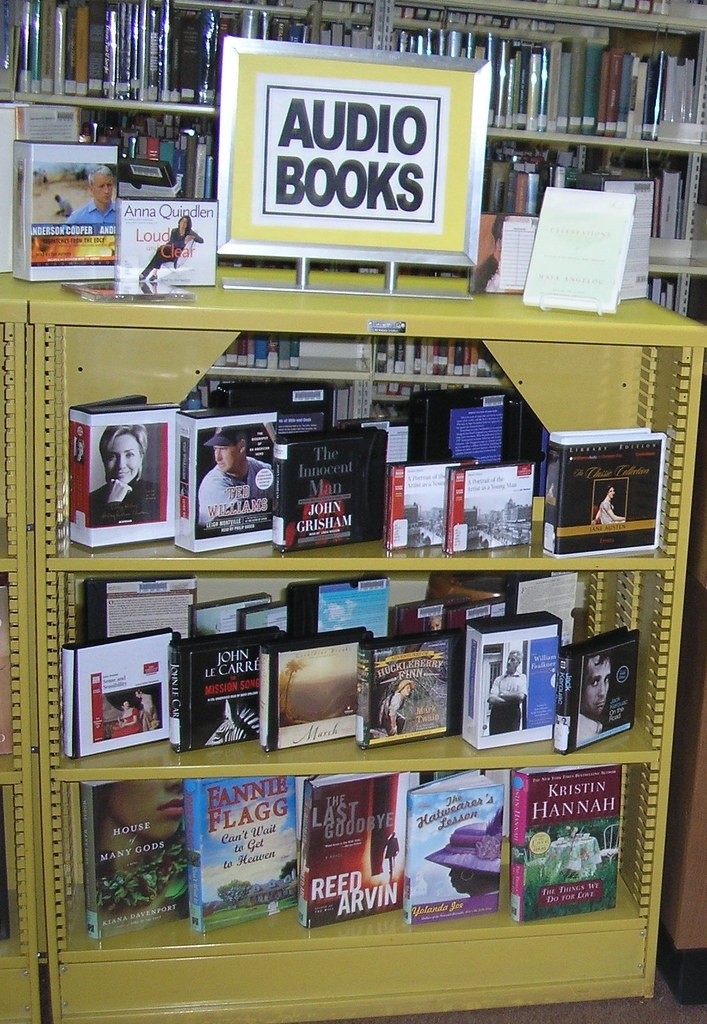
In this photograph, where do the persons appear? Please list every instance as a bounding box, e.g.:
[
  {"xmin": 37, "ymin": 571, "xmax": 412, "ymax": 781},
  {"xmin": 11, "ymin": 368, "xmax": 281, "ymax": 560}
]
[
  {"xmin": 73, "ymin": 439, "xmax": 84, "ymax": 463},
  {"xmin": 425, "ymin": 807, "xmax": 503, "ymax": 896},
  {"xmin": 134, "ymin": 690, "xmax": 159, "ymax": 731},
  {"xmin": 55, "ymin": 194, "xmax": 72, "ymax": 218},
  {"xmin": 63, "ymin": 166, "xmax": 116, "ymax": 224},
  {"xmin": 97, "ymin": 778, "xmax": 184, "ymax": 881},
  {"xmin": 90, "ymin": 424, "xmax": 162, "ymax": 524},
  {"xmin": 579, "ymin": 654, "xmax": 612, "ymax": 741},
  {"xmin": 379, "ymin": 680, "xmax": 414, "ymax": 737},
  {"xmin": 487, "ymin": 651, "xmax": 527, "ymax": 737},
  {"xmin": 428, "ymin": 615, "xmax": 441, "ymax": 631},
  {"xmin": 110, "ymin": 701, "xmax": 143, "ymax": 740},
  {"xmin": 591, "ymin": 485, "xmax": 626, "ymax": 525},
  {"xmin": 198, "ymin": 425, "xmax": 273, "ymax": 527},
  {"xmin": 139, "ymin": 215, "xmax": 203, "ymax": 280}
]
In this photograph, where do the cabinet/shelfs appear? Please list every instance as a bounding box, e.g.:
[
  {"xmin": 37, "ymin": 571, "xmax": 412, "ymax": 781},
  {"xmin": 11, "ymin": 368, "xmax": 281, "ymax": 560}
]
[
  {"xmin": 0, "ymin": 269, "xmax": 706, "ymax": 1024},
  {"xmin": 0, "ymin": 0, "xmax": 707, "ymax": 315}
]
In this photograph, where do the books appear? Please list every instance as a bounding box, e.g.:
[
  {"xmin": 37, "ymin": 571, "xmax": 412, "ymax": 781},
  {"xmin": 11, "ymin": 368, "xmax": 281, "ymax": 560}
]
[{"xmin": 0, "ymin": 0, "xmax": 707, "ymax": 940}]
